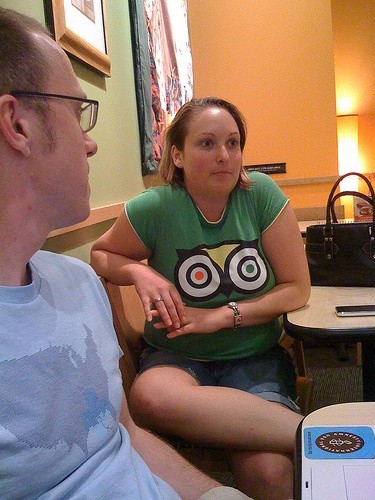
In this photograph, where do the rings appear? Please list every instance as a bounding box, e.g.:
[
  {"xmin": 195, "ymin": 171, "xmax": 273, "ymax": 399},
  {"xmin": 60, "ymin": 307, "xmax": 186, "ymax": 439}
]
[{"xmin": 152, "ymin": 298, "xmax": 163, "ymax": 304}]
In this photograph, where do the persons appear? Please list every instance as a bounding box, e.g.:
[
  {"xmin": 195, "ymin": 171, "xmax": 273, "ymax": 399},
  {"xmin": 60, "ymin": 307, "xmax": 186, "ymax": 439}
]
[
  {"xmin": 0, "ymin": 8, "xmax": 251, "ymax": 500},
  {"xmin": 91, "ymin": 96, "xmax": 312, "ymax": 500}
]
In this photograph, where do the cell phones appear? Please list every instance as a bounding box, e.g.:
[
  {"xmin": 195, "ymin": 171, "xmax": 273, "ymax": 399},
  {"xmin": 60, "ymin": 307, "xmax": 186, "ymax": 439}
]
[{"xmin": 335, "ymin": 305, "xmax": 375, "ymax": 317}]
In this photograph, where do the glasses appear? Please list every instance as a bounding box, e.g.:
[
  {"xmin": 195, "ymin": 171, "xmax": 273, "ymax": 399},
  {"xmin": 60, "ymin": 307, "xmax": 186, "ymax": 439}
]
[{"xmin": 10, "ymin": 90, "xmax": 99, "ymax": 133}]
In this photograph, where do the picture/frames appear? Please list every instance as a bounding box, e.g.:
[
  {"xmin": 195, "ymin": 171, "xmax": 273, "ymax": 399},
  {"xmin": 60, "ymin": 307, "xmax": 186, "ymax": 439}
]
[{"xmin": 43, "ymin": 0, "xmax": 112, "ymax": 78}]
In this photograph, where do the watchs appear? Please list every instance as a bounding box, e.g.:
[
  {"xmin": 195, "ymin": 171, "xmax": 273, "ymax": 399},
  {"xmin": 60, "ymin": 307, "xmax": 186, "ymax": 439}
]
[{"xmin": 228, "ymin": 302, "xmax": 242, "ymax": 333}]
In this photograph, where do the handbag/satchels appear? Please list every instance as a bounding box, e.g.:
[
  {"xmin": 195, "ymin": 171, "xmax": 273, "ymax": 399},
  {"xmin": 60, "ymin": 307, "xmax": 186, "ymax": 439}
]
[{"xmin": 305, "ymin": 173, "xmax": 374, "ymax": 288}]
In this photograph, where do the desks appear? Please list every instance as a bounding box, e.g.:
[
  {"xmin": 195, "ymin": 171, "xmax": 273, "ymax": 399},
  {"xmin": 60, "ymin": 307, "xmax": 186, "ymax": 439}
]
[{"xmin": 283, "ymin": 219, "xmax": 375, "ymax": 500}]
